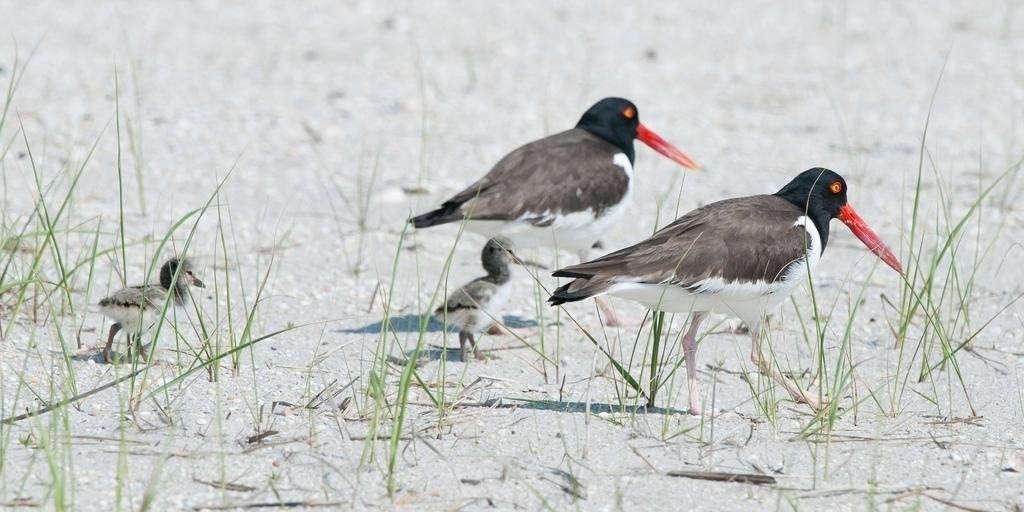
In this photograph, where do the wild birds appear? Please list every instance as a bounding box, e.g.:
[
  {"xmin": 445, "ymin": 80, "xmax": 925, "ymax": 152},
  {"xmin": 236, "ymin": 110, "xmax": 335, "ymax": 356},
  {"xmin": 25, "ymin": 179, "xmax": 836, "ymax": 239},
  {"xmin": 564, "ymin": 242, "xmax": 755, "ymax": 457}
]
[
  {"xmin": 547, "ymin": 168, "xmax": 905, "ymax": 416},
  {"xmin": 434, "ymin": 235, "xmax": 549, "ymax": 363},
  {"xmin": 95, "ymin": 256, "xmax": 206, "ymax": 366},
  {"xmin": 407, "ymin": 98, "xmax": 700, "ymax": 329}
]
